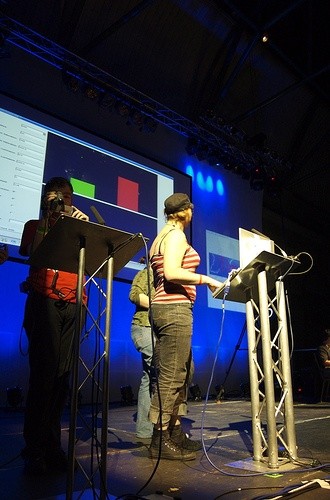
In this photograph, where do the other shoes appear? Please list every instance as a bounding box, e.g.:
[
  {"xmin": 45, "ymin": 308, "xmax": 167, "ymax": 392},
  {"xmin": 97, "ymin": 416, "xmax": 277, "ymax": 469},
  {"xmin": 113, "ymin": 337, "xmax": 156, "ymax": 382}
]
[{"xmin": 28, "ymin": 442, "xmax": 66, "ymax": 463}]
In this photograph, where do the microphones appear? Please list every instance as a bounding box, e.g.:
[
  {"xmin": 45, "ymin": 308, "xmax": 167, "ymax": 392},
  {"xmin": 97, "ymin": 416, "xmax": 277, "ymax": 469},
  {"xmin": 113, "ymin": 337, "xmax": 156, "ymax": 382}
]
[
  {"xmin": 90, "ymin": 205, "xmax": 105, "ymax": 227},
  {"xmin": 252, "ymin": 228, "xmax": 287, "ymax": 258}
]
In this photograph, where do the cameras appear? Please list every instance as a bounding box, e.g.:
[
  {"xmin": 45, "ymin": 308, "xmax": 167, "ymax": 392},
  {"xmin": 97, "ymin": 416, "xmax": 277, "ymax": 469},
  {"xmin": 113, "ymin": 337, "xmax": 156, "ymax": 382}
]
[{"xmin": 51, "ymin": 190, "xmax": 74, "ymax": 218}]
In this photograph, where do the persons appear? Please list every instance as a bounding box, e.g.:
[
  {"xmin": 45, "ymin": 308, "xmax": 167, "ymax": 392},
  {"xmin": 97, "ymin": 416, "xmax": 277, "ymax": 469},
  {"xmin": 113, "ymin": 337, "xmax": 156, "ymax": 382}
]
[
  {"xmin": 316, "ymin": 331, "xmax": 330, "ymax": 403},
  {"xmin": 20, "ymin": 177, "xmax": 88, "ymax": 467},
  {"xmin": 129, "ymin": 193, "xmax": 225, "ymax": 461}
]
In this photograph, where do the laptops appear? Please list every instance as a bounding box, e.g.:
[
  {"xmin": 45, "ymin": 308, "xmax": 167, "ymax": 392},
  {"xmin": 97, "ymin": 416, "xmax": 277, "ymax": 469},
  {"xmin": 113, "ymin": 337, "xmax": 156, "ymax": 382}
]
[{"xmin": 212, "ymin": 228, "xmax": 274, "ymax": 296}]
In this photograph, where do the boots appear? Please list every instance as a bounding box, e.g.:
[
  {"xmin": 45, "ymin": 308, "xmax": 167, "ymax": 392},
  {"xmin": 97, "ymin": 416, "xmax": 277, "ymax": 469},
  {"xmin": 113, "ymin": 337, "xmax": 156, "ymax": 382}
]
[
  {"xmin": 169, "ymin": 424, "xmax": 203, "ymax": 452},
  {"xmin": 149, "ymin": 427, "xmax": 197, "ymax": 461}
]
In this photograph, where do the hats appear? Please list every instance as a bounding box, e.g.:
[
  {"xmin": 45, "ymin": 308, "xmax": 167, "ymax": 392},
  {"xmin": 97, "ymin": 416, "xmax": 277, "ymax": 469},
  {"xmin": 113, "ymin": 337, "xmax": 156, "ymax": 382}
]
[{"xmin": 164, "ymin": 193, "xmax": 190, "ymax": 212}]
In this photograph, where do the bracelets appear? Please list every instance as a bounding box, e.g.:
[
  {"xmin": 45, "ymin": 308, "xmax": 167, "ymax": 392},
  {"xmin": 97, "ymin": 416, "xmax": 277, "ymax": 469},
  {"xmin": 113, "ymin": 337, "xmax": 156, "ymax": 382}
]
[
  {"xmin": 35, "ymin": 226, "xmax": 48, "ymax": 236},
  {"xmin": 199, "ymin": 274, "xmax": 202, "ymax": 284}
]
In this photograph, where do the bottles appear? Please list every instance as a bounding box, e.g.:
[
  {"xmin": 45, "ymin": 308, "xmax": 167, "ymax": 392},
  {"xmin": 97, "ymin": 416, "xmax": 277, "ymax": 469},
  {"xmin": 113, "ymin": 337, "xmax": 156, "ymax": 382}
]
[{"xmin": 49, "ymin": 191, "xmax": 64, "ymax": 228}]
